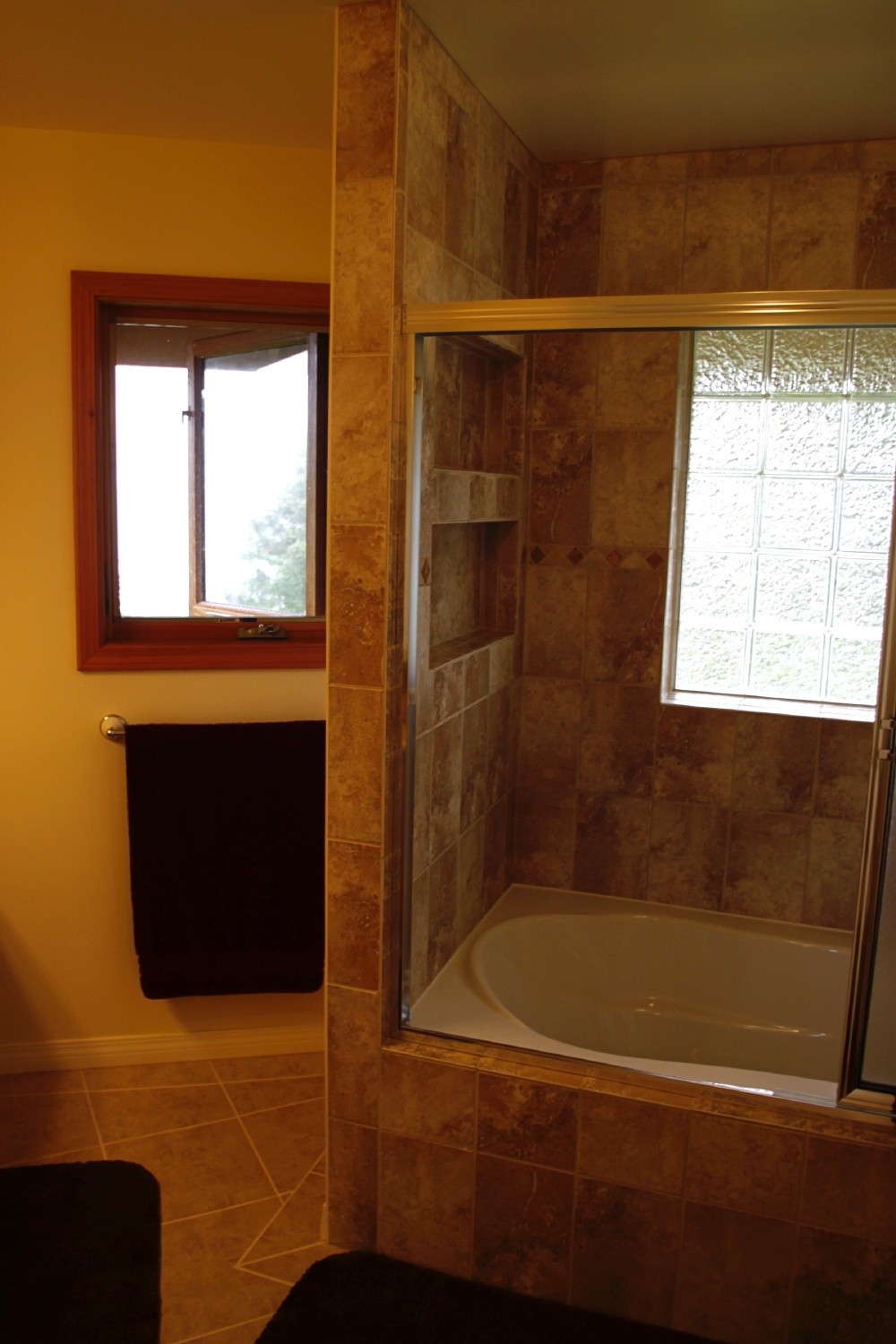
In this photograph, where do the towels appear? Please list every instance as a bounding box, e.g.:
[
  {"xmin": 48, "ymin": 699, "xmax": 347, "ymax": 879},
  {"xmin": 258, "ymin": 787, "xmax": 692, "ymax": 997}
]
[{"xmin": 121, "ymin": 719, "xmax": 330, "ymax": 1002}]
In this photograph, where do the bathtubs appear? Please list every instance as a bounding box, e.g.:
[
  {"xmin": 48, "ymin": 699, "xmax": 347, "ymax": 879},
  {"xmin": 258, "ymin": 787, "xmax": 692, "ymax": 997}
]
[{"xmin": 410, "ymin": 882, "xmax": 896, "ymax": 1120}]
